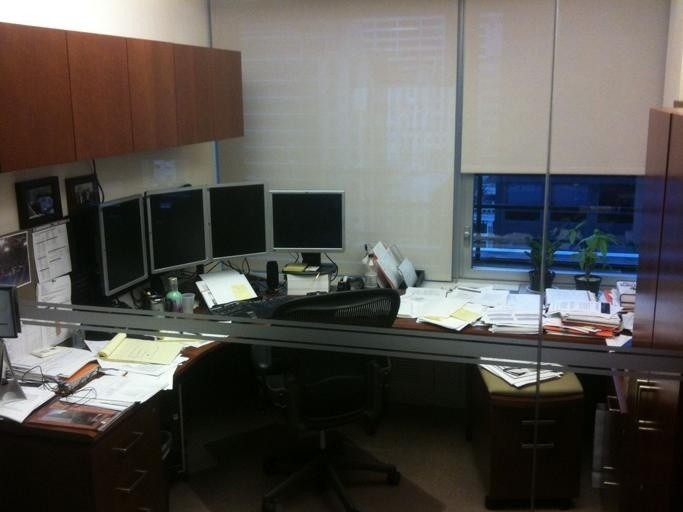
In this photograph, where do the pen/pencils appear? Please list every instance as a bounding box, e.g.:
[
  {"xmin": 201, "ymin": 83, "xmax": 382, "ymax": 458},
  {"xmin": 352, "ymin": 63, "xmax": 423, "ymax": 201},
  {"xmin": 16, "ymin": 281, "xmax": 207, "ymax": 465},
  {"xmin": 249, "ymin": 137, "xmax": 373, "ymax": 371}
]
[{"xmin": 365, "ymin": 245, "xmax": 368, "ymax": 256}]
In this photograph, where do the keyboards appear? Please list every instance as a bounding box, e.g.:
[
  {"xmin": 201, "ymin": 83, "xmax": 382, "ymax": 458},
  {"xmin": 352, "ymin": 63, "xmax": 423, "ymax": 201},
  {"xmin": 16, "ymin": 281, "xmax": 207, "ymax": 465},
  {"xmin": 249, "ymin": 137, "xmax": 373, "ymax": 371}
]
[{"xmin": 208, "ymin": 294, "xmax": 308, "ymax": 323}]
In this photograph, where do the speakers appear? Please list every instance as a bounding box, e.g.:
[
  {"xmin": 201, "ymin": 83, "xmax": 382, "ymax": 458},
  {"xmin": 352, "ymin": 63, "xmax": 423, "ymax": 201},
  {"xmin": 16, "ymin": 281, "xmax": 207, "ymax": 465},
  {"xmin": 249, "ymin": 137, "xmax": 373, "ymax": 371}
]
[{"xmin": 264, "ymin": 262, "xmax": 280, "ymax": 288}]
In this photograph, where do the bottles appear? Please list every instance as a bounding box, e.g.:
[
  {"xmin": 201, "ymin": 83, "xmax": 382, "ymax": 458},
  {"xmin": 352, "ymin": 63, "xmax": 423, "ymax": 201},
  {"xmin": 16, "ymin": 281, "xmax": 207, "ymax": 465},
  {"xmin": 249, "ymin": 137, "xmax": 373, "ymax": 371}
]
[
  {"xmin": 165, "ymin": 276, "xmax": 182, "ymax": 311},
  {"xmin": 365, "ymin": 254, "xmax": 376, "ymax": 287}
]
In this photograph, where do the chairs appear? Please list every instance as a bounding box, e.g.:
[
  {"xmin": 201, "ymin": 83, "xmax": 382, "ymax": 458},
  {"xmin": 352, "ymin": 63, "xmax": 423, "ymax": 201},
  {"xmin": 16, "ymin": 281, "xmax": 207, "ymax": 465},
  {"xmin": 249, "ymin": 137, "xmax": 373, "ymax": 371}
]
[{"xmin": 262, "ymin": 288, "xmax": 401, "ymax": 512}]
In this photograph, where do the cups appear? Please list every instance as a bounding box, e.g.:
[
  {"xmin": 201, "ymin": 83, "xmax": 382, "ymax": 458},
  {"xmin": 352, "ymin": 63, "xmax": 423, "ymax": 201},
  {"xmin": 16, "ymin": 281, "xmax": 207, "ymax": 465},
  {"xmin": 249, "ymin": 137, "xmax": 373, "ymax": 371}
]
[
  {"xmin": 182, "ymin": 293, "xmax": 195, "ymax": 313},
  {"xmin": 151, "ymin": 295, "xmax": 164, "ymax": 311}
]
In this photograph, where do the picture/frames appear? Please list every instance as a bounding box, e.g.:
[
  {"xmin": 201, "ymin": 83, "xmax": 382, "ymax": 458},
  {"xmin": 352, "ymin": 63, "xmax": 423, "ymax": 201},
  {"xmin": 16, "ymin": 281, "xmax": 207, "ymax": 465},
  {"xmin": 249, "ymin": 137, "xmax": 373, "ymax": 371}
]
[
  {"xmin": 0, "ymin": 231, "xmax": 31, "ymax": 288},
  {"xmin": 15, "ymin": 176, "xmax": 63, "ymax": 228},
  {"xmin": 65, "ymin": 175, "xmax": 100, "ymax": 216}
]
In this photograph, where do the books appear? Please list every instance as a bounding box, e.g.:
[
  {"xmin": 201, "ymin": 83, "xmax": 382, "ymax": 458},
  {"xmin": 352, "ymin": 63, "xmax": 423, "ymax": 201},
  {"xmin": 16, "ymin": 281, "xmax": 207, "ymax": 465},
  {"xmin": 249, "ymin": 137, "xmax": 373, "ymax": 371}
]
[
  {"xmin": 12, "ymin": 346, "xmax": 98, "ymax": 390},
  {"xmin": 97, "ymin": 332, "xmax": 182, "ymax": 365},
  {"xmin": 616, "ymin": 281, "xmax": 636, "ymax": 307},
  {"xmin": 476, "ymin": 355, "xmax": 564, "ymax": 387},
  {"xmin": 397, "ymin": 286, "xmax": 543, "ymax": 335},
  {"xmin": 543, "ymin": 287, "xmax": 620, "ymax": 338}
]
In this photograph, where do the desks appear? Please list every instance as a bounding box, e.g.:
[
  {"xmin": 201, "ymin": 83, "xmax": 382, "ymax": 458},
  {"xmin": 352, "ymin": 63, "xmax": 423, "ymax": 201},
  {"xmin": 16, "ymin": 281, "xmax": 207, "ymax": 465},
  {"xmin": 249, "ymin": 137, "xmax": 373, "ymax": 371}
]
[{"xmin": 0, "ymin": 288, "xmax": 630, "ymax": 512}]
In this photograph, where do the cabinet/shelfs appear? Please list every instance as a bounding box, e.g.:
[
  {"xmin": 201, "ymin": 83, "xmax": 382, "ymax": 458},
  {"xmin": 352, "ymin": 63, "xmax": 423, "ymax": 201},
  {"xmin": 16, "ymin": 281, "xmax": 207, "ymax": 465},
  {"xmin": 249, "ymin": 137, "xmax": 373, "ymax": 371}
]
[
  {"xmin": 67, "ymin": 30, "xmax": 178, "ymax": 161},
  {"xmin": 474, "ymin": 362, "xmax": 584, "ymax": 512},
  {"xmin": 0, "ymin": 22, "xmax": 77, "ymax": 173},
  {"xmin": 174, "ymin": 43, "xmax": 244, "ymax": 146},
  {"xmin": 623, "ymin": 109, "xmax": 683, "ymax": 512}
]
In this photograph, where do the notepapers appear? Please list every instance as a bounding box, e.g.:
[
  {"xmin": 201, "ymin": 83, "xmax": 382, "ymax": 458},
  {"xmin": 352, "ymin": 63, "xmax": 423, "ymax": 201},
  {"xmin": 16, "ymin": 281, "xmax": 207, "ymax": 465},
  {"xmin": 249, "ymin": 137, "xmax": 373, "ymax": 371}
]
[{"xmin": 283, "ymin": 263, "xmax": 308, "ymax": 272}]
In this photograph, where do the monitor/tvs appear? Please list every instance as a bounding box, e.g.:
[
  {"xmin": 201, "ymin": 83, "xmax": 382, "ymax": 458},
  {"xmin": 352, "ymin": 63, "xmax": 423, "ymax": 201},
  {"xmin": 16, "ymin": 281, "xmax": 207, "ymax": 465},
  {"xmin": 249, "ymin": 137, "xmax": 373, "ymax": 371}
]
[
  {"xmin": 96, "ymin": 194, "xmax": 147, "ymax": 298},
  {"xmin": 208, "ymin": 182, "xmax": 267, "ymax": 261},
  {"xmin": 144, "ymin": 185, "xmax": 207, "ymax": 275},
  {"xmin": 268, "ymin": 188, "xmax": 344, "ymax": 274}
]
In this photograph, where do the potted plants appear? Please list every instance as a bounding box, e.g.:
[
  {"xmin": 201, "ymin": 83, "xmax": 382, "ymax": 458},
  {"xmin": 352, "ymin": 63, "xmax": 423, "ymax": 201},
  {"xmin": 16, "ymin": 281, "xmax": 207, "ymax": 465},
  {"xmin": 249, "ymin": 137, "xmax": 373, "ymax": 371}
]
[
  {"xmin": 523, "ymin": 235, "xmax": 561, "ymax": 291},
  {"xmin": 567, "ymin": 223, "xmax": 618, "ymax": 296}
]
